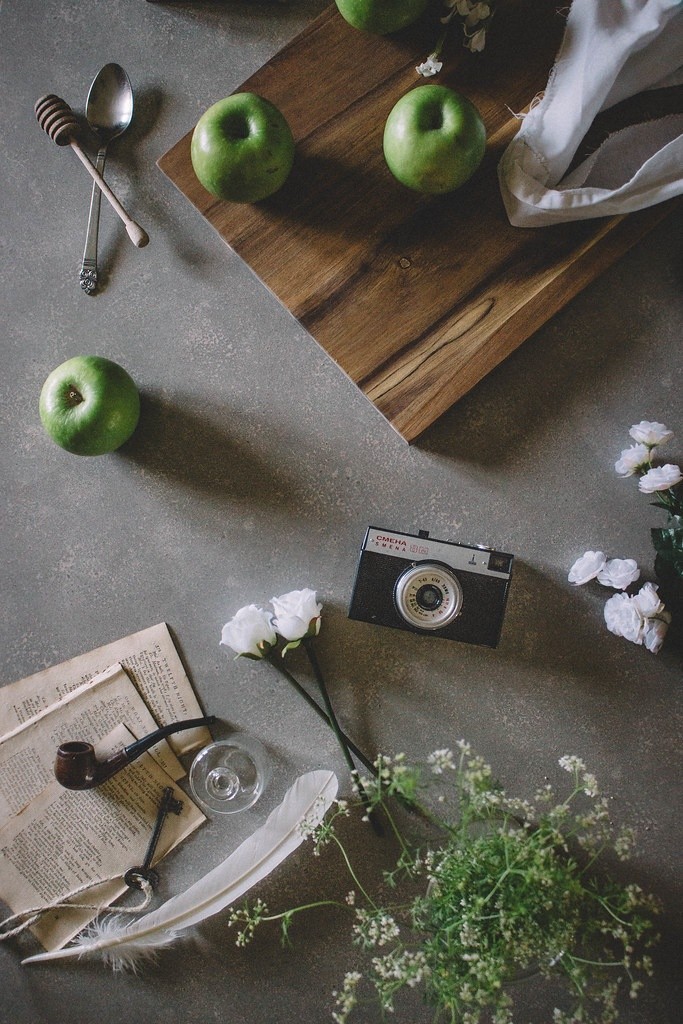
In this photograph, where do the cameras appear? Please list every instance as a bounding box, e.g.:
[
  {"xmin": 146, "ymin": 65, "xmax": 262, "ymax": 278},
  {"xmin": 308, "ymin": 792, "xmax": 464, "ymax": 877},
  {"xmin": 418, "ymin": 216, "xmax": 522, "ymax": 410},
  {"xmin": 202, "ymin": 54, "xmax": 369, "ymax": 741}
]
[{"xmin": 347, "ymin": 525, "xmax": 515, "ymax": 649}]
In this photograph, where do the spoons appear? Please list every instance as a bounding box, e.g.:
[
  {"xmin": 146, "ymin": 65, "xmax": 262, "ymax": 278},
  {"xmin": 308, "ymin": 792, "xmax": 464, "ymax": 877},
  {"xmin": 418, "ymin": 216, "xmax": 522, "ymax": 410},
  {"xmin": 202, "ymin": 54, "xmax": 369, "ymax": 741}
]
[{"xmin": 79, "ymin": 63, "xmax": 134, "ymax": 296}]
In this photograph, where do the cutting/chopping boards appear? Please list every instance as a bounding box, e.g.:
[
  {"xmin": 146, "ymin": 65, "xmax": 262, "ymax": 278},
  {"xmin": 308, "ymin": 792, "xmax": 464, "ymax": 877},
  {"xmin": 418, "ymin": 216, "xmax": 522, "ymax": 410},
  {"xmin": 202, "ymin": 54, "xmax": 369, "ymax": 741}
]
[{"xmin": 156, "ymin": 0, "xmax": 682, "ymax": 446}]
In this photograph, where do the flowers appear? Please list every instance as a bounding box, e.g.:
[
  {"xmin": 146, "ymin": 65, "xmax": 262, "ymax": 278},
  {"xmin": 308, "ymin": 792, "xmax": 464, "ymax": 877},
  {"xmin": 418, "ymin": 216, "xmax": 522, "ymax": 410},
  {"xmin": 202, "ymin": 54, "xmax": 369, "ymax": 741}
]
[
  {"xmin": 566, "ymin": 419, "xmax": 682, "ymax": 656},
  {"xmin": 217, "ymin": 585, "xmax": 416, "ymax": 834},
  {"xmin": 411, "ymin": 0, "xmax": 501, "ymax": 79},
  {"xmin": 211, "ymin": 733, "xmax": 668, "ymax": 1023}
]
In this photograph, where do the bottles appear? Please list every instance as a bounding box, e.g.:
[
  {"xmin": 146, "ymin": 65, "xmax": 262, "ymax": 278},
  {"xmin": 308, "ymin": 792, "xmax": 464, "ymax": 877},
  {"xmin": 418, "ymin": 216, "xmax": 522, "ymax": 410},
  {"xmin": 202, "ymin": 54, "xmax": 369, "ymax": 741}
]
[{"xmin": 189, "ymin": 734, "xmax": 272, "ymax": 815}]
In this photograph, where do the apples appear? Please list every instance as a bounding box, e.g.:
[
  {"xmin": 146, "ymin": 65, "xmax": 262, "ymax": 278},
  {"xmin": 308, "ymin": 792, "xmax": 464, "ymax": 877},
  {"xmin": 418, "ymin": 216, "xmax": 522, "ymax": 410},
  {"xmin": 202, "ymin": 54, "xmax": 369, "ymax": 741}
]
[
  {"xmin": 384, "ymin": 85, "xmax": 487, "ymax": 194},
  {"xmin": 332, "ymin": 0, "xmax": 429, "ymax": 35},
  {"xmin": 39, "ymin": 355, "xmax": 139, "ymax": 457},
  {"xmin": 191, "ymin": 92, "xmax": 292, "ymax": 204}
]
